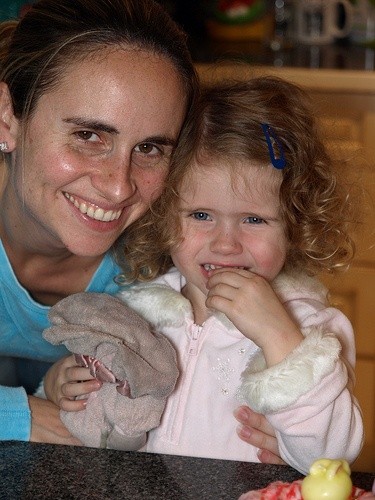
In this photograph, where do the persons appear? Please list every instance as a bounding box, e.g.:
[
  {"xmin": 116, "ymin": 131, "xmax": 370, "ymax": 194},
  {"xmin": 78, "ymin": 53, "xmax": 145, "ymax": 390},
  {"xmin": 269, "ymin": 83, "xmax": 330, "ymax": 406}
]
[
  {"xmin": 33, "ymin": 75, "xmax": 367, "ymax": 477},
  {"xmin": 0, "ymin": 1, "xmax": 290, "ymax": 465}
]
